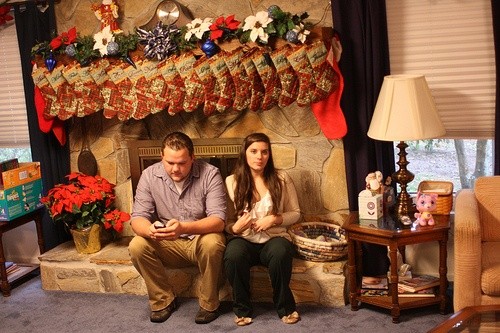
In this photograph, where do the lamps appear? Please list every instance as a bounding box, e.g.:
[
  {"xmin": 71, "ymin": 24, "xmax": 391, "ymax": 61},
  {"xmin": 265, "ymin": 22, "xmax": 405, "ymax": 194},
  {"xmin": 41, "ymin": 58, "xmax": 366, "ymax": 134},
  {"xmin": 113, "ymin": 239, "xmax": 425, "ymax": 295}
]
[{"xmin": 366, "ymin": 74, "xmax": 446, "ymax": 226}]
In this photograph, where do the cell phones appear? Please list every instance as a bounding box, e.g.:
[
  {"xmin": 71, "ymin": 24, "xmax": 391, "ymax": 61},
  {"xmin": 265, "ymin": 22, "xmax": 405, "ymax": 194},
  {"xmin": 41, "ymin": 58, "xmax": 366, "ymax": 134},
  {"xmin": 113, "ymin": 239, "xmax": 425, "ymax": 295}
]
[{"xmin": 154, "ymin": 224, "xmax": 166, "ymax": 235}]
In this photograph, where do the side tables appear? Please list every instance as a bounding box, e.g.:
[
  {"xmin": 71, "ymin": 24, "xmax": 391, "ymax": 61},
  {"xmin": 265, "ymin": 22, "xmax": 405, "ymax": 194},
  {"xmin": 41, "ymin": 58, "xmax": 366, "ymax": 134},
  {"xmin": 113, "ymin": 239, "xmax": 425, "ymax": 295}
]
[{"xmin": 342, "ymin": 211, "xmax": 450, "ymax": 324}]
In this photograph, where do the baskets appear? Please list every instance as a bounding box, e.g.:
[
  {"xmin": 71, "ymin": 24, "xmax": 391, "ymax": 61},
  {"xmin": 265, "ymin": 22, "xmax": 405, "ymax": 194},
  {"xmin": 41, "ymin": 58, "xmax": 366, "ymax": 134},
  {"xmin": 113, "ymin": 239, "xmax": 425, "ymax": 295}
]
[
  {"xmin": 418, "ymin": 180, "xmax": 453, "ymax": 215},
  {"xmin": 288, "ymin": 221, "xmax": 348, "ymax": 263}
]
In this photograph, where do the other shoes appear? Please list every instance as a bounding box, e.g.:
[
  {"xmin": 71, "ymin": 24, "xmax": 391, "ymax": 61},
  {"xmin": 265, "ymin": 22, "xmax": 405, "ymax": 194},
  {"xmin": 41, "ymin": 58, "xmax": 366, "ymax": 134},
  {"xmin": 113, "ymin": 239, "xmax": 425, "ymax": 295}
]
[
  {"xmin": 150, "ymin": 297, "xmax": 180, "ymax": 323},
  {"xmin": 195, "ymin": 301, "xmax": 225, "ymax": 324},
  {"xmin": 233, "ymin": 314, "xmax": 255, "ymax": 326},
  {"xmin": 280, "ymin": 309, "xmax": 301, "ymax": 324}
]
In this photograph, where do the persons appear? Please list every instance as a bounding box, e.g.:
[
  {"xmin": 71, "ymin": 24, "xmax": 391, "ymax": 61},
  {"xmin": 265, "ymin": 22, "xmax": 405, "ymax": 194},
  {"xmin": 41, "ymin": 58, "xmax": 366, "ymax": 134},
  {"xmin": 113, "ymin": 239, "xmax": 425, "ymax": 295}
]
[
  {"xmin": 223, "ymin": 133, "xmax": 301, "ymax": 325},
  {"xmin": 128, "ymin": 131, "xmax": 229, "ymax": 323}
]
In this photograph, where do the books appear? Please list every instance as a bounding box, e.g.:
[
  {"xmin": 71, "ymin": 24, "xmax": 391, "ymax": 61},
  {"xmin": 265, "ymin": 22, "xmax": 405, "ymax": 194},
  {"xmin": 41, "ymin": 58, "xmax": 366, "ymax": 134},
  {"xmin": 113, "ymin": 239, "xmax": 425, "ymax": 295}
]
[
  {"xmin": 361, "ymin": 273, "xmax": 440, "ymax": 297},
  {"xmin": 5, "ymin": 261, "xmax": 21, "ymax": 274}
]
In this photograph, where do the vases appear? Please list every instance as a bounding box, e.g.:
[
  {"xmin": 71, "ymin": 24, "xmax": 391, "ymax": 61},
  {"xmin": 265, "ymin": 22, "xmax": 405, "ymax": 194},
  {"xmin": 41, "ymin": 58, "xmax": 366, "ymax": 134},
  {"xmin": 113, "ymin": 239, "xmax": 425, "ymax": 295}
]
[{"xmin": 70, "ymin": 224, "xmax": 101, "ymax": 254}]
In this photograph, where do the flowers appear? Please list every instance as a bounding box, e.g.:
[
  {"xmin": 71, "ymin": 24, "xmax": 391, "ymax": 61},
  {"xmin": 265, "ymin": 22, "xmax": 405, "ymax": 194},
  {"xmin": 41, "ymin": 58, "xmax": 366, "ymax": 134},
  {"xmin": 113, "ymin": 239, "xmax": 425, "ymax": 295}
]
[
  {"xmin": 31, "ymin": 7, "xmax": 311, "ymax": 62},
  {"xmin": 40, "ymin": 172, "xmax": 131, "ymax": 233}
]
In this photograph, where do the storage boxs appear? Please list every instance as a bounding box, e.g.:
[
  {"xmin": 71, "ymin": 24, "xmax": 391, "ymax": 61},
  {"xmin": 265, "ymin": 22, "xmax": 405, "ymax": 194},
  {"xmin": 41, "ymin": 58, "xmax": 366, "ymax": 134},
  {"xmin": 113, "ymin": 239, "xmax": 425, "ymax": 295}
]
[
  {"xmin": 418, "ymin": 181, "xmax": 453, "ymax": 216},
  {"xmin": 0, "ymin": 158, "xmax": 44, "ymax": 221},
  {"xmin": 358, "ymin": 194, "xmax": 383, "ymax": 219}
]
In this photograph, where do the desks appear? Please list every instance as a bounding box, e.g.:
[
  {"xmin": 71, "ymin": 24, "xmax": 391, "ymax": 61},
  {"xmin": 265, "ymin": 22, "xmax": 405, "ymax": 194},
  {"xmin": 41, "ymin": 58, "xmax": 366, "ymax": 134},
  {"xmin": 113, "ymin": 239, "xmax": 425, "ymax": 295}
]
[
  {"xmin": 0, "ymin": 204, "xmax": 46, "ymax": 297},
  {"xmin": 425, "ymin": 304, "xmax": 500, "ymax": 333}
]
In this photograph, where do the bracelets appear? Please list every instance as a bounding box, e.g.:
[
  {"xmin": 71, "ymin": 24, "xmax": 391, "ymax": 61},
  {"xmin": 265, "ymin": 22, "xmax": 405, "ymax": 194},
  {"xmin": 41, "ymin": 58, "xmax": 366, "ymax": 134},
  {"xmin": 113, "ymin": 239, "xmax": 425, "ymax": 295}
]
[{"xmin": 273, "ymin": 215, "xmax": 279, "ymax": 226}]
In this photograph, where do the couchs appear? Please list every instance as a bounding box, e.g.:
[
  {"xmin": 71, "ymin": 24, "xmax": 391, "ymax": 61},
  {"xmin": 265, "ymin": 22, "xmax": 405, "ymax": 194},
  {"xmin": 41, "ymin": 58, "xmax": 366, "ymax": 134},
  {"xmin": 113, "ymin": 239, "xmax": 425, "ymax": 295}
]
[{"xmin": 453, "ymin": 176, "xmax": 500, "ymax": 333}]
